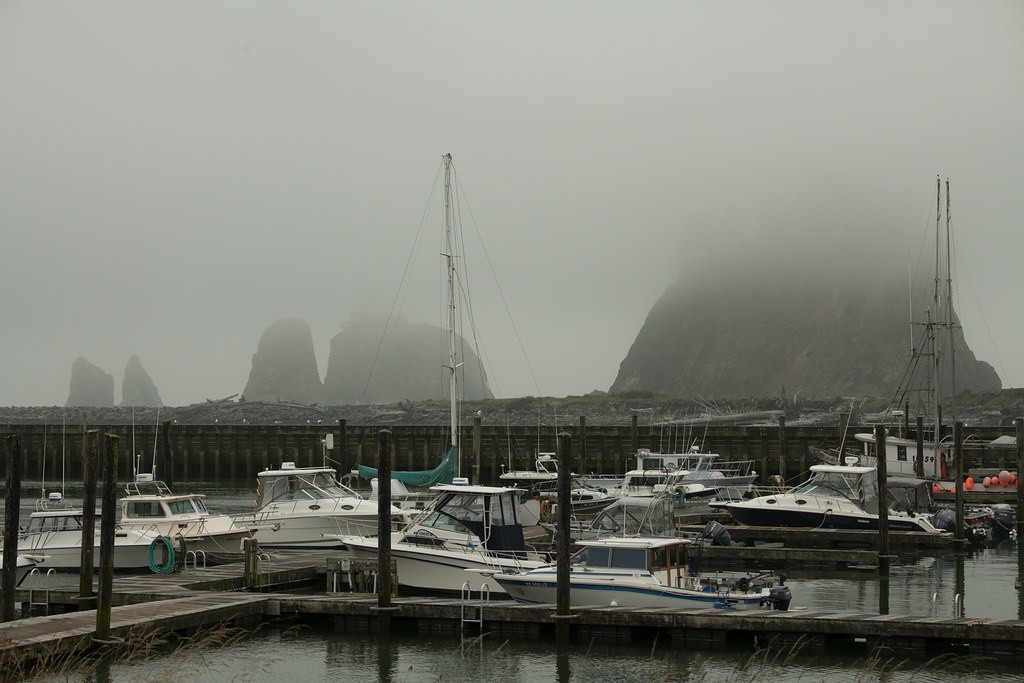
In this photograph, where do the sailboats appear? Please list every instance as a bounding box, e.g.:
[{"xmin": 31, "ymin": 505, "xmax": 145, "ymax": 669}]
[
  {"xmin": 463, "ymin": 456, "xmax": 793, "ymax": 610},
  {"xmin": 334, "ymin": 151, "xmax": 544, "ymax": 529}
]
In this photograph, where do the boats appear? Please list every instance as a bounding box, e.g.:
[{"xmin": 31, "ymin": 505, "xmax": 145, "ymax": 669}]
[
  {"xmin": 0, "ymin": 525, "xmax": 60, "ymax": 588},
  {"xmin": 497, "ymin": 174, "xmax": 1024, "ymax": 544},
  {"xmin": 708, "ymin": 396, "xmax": 989, "ymax": 543},
  {"xmin": 0, "ymin": 395, "xmax": 286, "ymax": 572},
  {"xmin": 322, "ymin": 484, "xmax": 554, "ymax": 600},
  {"xmin": 228, "ymin": 431, "xmax": 433, "ymax": 550}
]
